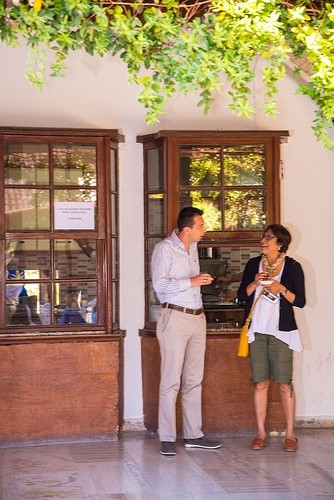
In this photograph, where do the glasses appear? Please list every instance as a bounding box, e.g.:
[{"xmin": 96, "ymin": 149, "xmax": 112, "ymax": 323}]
[{"xmin": 260, "ymin": 233, "xmax": 278, "ymax": 240}]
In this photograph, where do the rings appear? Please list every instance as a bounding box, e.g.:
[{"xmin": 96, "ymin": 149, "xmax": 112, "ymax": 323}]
[{"xmin": 204, "ymin": 280, "xmax": 206, "ymax": 283}]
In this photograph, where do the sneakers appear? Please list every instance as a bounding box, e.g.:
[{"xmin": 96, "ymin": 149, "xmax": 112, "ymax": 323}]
[
  {"xmin": 159, "ymin": 439, "xmax": 177, "ymax": 455},
  {"xmin": 184, "ymin": 437, "xmax": 222, "ymax": 449}
]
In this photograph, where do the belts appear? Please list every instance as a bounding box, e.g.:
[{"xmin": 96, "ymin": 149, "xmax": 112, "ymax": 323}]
[{"xmin": 162, "ymin": 303, "xmax": 204, "ymax": 315}]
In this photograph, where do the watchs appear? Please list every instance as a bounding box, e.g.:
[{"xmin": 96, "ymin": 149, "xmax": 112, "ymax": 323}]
[{"xmin": 281, "ymin": 289, "xmax": 288, "ymax": 296}]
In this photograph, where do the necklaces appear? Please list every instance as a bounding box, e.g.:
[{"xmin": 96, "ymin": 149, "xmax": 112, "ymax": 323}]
[{"xmin": 262, "ymin": 250, "xmax": 285, "ymax": 271}]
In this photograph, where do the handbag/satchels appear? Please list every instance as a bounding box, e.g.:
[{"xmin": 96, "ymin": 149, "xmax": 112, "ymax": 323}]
[{"xmin": 238, "ymin": 324, "xmax": 250, "ymax": 358}]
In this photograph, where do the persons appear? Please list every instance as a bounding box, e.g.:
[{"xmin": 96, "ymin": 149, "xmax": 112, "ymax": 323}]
[
  {"xmin": 151, "ymin": 207, "xmax": 222, "ymax": 455},
  {"xmin": 237, "ymin": 223, "xmax": 307, "ymax": 452}
]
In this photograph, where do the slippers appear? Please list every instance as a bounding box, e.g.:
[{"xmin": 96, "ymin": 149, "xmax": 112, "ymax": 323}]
[
  {"xmin": 252, "ymin": 438, "xmax": 266, "ymax": 450},
  {"xmin": 284, "ymin": 435, "xmax": 298, "ymax": 451}
]
